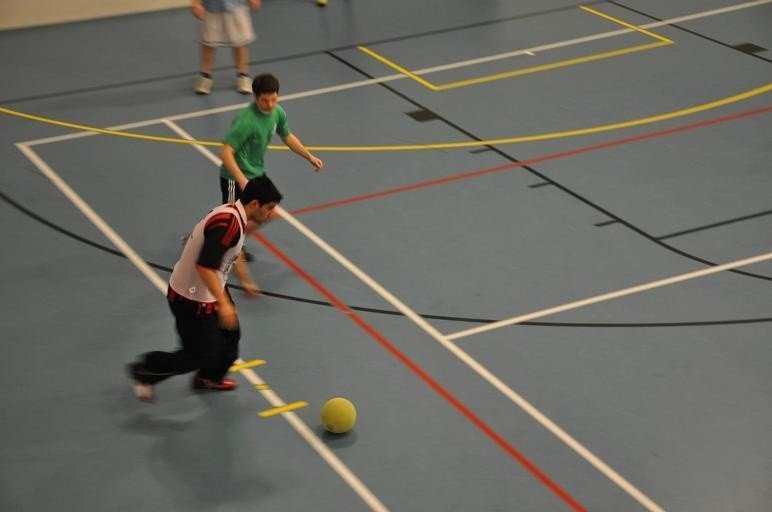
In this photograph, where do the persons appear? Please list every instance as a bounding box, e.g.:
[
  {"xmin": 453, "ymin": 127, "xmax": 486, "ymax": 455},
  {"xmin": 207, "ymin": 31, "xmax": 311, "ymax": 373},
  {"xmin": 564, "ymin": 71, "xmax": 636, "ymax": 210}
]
[
  {"xmin": 176, "ymin": 72, "xmax": 324, "ymax": 262},
  {"xmin": 121, "ymin": 175, "xmax": 285, "ymax": 401},
  {"xmin": 189, "ymin": 0, "xmax": 264, "ymax": 96}
]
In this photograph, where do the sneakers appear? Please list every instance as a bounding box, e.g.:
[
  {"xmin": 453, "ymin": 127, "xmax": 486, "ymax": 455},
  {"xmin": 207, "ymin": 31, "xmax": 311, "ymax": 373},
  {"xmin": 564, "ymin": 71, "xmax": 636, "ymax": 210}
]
[
  {"xmin": 244, "ymin": 251, "xmax": 255, "ymax": 262},
  {"xmin": 125, "ymin": 362, "xmax": 152, "ymax": 401},
  {"xmin": 237, "ymin": 76, "xmax": 252, "ymax": 94},
  {"xmin": 193, "ymin": 379, "xmax": 238, "ymax": 390},
  {"xmin": 194, "ymin": 75, "xmax": 213, "ymax": 94}
]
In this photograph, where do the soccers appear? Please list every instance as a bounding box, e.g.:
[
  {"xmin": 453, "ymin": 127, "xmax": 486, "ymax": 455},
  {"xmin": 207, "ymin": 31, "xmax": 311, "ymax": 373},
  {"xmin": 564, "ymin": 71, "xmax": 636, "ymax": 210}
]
[{"xmin": 320, "ymin": 398, "xmax": 357, "ymax": 435}]
